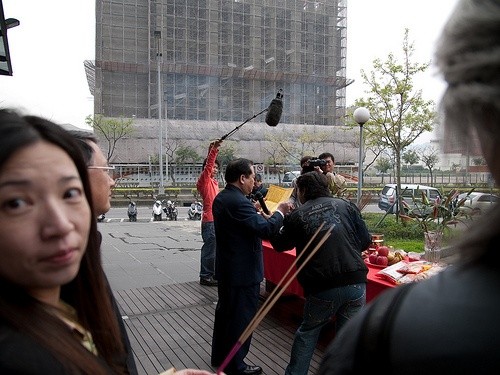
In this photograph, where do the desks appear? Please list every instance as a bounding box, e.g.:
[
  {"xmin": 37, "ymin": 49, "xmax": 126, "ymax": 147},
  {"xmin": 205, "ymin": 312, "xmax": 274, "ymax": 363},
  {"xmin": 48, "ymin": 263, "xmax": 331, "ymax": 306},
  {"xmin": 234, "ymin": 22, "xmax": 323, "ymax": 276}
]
[{"xmin": 261, "ymin": 239, "xmax": 447, "ymax": 321}]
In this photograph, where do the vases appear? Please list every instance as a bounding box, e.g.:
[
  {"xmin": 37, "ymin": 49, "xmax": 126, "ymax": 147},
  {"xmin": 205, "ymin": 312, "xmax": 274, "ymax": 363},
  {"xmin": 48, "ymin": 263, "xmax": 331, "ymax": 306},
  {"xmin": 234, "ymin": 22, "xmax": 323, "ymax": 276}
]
[{"xmin": 425, "ymin": 232, "xmax": 444, "ymax": 262}]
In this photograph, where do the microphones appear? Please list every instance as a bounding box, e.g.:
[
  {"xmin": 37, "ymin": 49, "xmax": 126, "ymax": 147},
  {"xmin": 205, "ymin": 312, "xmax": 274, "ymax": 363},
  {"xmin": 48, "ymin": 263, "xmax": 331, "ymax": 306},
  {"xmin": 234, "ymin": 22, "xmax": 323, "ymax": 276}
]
[{"xmin": 265, "ymin": 88, "xmax": 284, "ymax": 127}]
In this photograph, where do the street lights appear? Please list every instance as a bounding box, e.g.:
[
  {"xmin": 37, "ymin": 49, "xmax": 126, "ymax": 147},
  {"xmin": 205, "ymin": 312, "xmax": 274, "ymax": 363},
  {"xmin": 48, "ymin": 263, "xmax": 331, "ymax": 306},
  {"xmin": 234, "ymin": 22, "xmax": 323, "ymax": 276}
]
[{"xmin": 352, "ymin": 107, "xmax": 371, "ymax": 208}]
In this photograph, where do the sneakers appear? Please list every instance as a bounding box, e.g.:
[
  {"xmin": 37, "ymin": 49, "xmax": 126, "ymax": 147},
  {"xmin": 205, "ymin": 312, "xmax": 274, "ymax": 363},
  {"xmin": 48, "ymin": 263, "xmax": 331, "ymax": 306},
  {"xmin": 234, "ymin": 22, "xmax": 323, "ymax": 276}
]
[{"xmin": 200, "ymin": 275, "xmax": 218, "ymax": 286}]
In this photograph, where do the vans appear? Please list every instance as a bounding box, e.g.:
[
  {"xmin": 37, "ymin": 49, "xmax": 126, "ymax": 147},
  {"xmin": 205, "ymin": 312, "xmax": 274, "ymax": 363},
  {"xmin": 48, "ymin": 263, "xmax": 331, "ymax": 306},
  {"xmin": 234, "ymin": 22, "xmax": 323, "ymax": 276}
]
[{"xmin": 378, "ymin": 184, "xmax": 451, "ymax": 219}]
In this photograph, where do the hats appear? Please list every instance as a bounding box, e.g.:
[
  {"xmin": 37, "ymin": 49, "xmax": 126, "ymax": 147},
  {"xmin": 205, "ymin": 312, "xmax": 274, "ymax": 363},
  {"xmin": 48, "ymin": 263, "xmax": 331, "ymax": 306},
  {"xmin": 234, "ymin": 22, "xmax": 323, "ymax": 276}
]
[
  {"xmin": 434, "ymin": 0, "xmax": 500, "ymax": 154},
  {"xmin": 255, "ymin": 173, "xmax": 262, "ymax": 180}
]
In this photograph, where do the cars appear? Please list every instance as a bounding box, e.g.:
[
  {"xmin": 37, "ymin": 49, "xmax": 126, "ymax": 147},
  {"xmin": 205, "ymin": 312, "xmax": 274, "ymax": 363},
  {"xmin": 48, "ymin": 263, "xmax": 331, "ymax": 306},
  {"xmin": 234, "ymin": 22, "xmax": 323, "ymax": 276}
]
[
  {"xmin": 452, "ymin": 192, "xmax": 500, "ymax": 216},
  {"xmin": 282, "ymin": 171, "xmax": 302, "ymax": 187}
]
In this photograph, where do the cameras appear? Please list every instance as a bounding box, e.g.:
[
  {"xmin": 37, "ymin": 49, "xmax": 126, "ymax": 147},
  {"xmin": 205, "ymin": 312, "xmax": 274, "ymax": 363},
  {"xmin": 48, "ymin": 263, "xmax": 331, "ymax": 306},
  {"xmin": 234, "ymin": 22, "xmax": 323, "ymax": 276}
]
[{"xmin": 306, "ymin": 157, "xmax": 326, "ymax": 174}]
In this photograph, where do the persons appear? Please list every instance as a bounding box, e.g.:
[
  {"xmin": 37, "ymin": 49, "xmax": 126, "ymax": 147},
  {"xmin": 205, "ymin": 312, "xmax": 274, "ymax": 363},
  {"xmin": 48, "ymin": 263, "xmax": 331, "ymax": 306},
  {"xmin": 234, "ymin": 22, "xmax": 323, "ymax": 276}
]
[
  {"xmin": 211, "ymin": 159, "xmax": 291, "ymax": 375},
  {"xmin": 290, "ymin": 152, "xmax": 347, "ymax": 212},
  {"xmin": 314, "ymin": 0, "xmax": 500, "ymax": 375},
  {"xmin": 195, "ymin": 141, "xmax": 222, "ymax": 286},
  {"xmin": 271, "ymin": 172, "xmax": 372, "ymax": 375},
  {"xmin": 0, "ymin": 108, "xmax": 215, "ymax": 375},
  {"xmin": 58, "ymin": 130, "xmax": 137, "ymax": 375},
  {"xmin": 248, "ymin": 174, "xmax": 268, "ymax": 205}
]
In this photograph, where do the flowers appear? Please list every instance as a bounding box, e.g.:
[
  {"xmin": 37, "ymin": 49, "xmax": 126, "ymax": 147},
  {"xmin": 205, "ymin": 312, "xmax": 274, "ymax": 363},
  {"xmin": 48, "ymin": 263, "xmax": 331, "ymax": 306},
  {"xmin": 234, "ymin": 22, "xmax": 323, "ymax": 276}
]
[{"xmin": 395, "ymin": 189, "xmax": 481, "ymax": 259}]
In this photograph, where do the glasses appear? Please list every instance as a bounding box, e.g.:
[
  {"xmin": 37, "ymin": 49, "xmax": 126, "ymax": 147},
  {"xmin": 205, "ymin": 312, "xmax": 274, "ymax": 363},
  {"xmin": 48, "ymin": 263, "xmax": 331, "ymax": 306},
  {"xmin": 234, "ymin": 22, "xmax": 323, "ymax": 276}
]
[
  {"xmin": 256, "ymin": 180, "xmax": 262, "ymax": 182},
  {"xmin": 88, "ymin": 163, "xmax": 117, "ymax": 179}
]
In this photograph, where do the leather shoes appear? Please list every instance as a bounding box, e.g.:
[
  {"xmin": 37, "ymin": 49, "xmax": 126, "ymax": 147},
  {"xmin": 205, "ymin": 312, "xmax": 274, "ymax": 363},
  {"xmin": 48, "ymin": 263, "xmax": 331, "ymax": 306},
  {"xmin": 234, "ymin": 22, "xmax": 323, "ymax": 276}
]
[{"xmin": 241, "ymin": 365, "xmax": 263, "ymax": 375}]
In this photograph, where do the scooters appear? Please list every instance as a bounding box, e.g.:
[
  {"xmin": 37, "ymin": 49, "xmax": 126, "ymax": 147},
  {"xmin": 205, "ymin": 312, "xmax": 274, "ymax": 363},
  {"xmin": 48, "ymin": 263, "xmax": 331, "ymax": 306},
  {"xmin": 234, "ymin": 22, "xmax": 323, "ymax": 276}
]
[
  {"xmin": 124, "ymin": 194, "xmax": 138, "ymax": 222},
  {"xmin": 153, "ymin": 197, "xmax": 180, "ymax": 221},
  {"xmin": 188, "ymin": 198, "xmax": 203, "ymax": 220}
]
